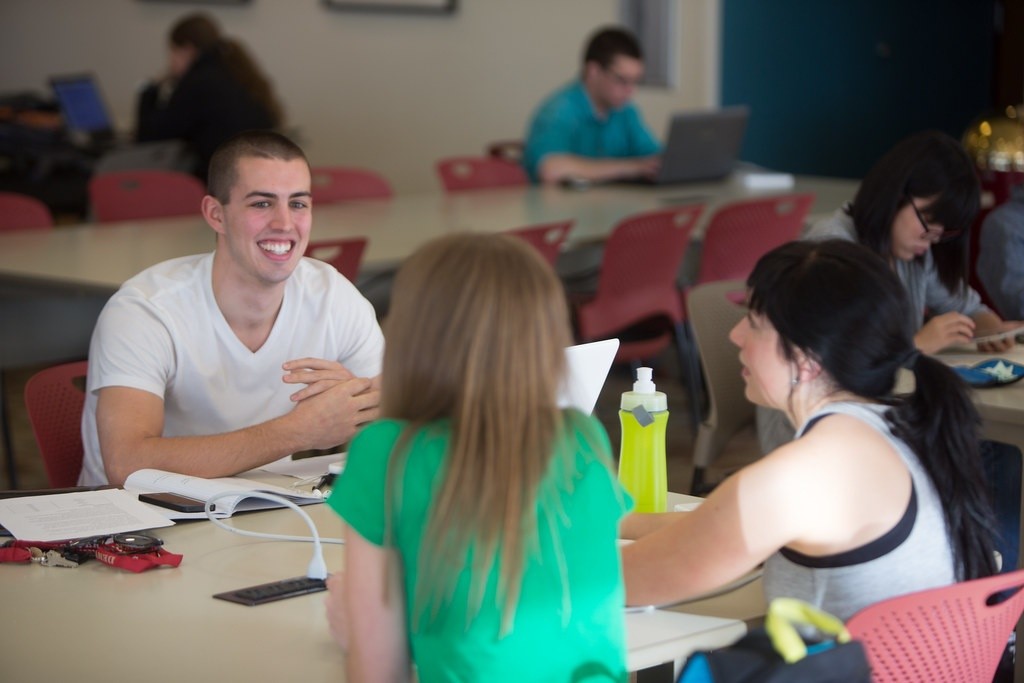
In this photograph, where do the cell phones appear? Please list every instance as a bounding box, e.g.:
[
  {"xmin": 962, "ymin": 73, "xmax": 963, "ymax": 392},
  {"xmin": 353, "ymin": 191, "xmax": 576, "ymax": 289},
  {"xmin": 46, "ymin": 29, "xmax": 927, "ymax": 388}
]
[{"xmin": 138, "ymin": 492, "xmax": 215, "ymax": 512}]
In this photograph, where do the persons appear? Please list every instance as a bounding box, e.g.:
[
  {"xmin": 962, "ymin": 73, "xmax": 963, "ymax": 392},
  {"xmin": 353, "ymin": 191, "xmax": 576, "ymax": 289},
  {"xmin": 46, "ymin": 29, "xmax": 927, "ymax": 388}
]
[
  {"xmin": 619, "ymin": 236, "xmax": 1000, "ymax": 643},
  {"xmin": 324, "ymin": 232, "xmax": 637, "ymax": 683},
  {"xmin": 134, "ymin": 13, "xmax": 282, "ymax": 180},
  {"xmin": 755, "ymin": 130, "xmax": 1024, "ymax": 653},
  {"xmin": 76, "ymin": 132, "xmax": 387, "ymax": 486},
  {"xmin": 523, "ymin": 27, "xmax": 665, "ymax": 183},
  {"xmin": 978, "ymin": 184, "xmax": 1024, "ymax": 320}
]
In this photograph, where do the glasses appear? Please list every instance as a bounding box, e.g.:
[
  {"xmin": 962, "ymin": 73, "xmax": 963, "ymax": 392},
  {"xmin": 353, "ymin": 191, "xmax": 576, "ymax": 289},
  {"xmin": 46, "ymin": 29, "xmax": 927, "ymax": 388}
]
[
  {"xmin": 906, "ymin": 192, "xmax": 962, "ymax": 243},
  {"xmin": 602, "ymin": 64, "xmax": 642, "ymax": 86}
]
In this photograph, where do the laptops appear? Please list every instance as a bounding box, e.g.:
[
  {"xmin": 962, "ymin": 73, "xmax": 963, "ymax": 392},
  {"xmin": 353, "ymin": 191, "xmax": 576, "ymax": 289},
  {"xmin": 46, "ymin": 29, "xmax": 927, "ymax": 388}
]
[
  {"xmin": 614, "ymin": 110, "xmax": 749, "ymax": 187},
  {"xmin": 51, "ymin": 72, "xmax": 131, "ymax": 145}
]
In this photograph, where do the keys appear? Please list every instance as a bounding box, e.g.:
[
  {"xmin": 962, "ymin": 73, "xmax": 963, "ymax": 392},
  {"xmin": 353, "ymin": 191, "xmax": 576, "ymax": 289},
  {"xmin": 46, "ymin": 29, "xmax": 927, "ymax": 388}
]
[{"xmin": 42, "ymin": 551, "xmax": 78, "ymax": 568}]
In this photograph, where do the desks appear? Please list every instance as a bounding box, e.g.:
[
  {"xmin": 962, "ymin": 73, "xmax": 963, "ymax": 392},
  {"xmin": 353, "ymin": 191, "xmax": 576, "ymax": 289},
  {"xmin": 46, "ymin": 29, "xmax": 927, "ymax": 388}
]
[
  {"xmin": 878, "ymin": 328, "xmax": 1024, "ymax": 451},
  {"xmin": 0, "ymin": 175, "xmax": 859, "ymax": 302},
  {"xmin": 0, "ymin": 467, "xmax": 769, "ymax": 683},
  {"xmin": 65, "ymin": 130, "xmax": 178, "ymax": 176}
]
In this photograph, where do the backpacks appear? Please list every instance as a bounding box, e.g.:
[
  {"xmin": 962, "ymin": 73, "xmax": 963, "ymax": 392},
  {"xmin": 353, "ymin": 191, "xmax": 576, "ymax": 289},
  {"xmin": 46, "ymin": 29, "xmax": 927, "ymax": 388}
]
[{"xmin": 678, "ymin": 598, "xmax": 872, "ymax": 683}]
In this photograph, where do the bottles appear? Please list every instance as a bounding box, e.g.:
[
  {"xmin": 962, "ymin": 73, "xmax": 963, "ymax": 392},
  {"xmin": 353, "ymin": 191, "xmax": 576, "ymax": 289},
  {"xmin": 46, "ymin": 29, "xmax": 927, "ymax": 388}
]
[{"xmin": 616, "ymin": 366, "xmax": 669, "ymax": 513}]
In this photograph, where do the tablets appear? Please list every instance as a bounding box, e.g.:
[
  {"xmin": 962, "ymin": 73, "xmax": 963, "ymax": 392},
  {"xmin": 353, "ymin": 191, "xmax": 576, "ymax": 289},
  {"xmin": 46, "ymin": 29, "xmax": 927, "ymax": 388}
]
[{"xmin": 966, "ymin": 326, "xmax": 1024, "ymax": 342}]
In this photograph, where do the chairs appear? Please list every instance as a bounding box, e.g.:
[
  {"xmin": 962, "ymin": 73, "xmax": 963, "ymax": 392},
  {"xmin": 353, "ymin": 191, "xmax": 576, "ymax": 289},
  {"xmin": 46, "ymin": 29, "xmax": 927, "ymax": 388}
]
[{"xmin": 0, "ymin": 118, "xmax": 1024, "ymax": 683}]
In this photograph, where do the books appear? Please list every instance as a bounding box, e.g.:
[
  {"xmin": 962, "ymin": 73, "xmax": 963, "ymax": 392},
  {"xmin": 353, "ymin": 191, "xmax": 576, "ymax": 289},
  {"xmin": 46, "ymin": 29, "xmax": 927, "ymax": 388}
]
[{"xmin": 121, "ymin": 469, "xmax": 324, "ymax": 520}]
